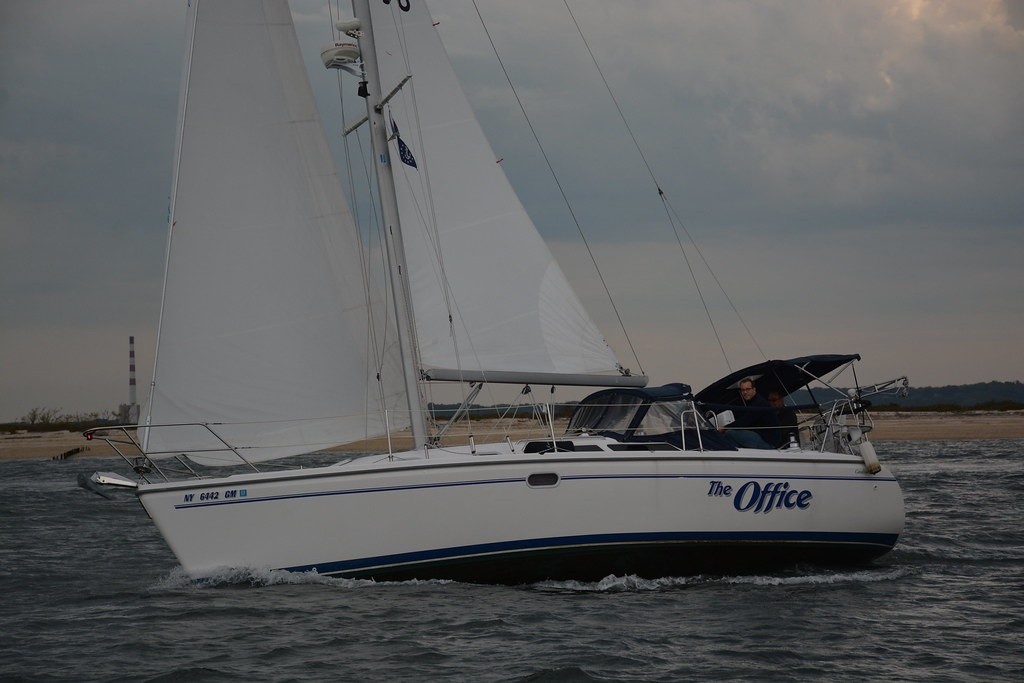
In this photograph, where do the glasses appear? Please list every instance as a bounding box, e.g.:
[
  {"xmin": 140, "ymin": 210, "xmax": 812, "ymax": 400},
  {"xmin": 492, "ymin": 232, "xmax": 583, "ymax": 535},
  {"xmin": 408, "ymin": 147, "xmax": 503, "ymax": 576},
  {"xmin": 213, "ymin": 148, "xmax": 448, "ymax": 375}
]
[
  {"xmin": 741, "ymin": 388, "xmax": 753, "ymax": 392},
  {"xmin": 767, "ymin": 398, "xmax": 780, "ymax": 402}
]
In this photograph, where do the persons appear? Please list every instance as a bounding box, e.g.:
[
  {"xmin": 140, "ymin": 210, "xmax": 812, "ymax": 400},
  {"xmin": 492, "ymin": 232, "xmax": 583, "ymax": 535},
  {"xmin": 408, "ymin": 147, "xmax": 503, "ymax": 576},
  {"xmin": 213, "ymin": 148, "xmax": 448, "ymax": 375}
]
[
  {"xmin": 767, "ymin": 389, "xmax": 800, "ymax": 450},
  {"xmin": 731, "ymin": 378, "xmax": 769, "ymax": 407}
]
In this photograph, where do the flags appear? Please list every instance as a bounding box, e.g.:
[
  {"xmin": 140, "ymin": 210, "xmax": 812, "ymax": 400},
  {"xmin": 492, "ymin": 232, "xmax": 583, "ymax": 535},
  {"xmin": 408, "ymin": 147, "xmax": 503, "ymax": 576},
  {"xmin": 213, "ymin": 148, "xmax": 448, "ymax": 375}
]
[{"xmin": 398, "ymin": 138, "xmax": 417, "ymax": 168}]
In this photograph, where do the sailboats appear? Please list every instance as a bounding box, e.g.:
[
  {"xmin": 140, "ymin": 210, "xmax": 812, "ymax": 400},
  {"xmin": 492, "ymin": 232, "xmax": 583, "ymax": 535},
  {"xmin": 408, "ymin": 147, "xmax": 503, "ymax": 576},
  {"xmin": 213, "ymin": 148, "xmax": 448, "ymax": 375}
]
[{"xmin": 77, "ymin": 1, "xmax": 909, "ymax": 591}]
